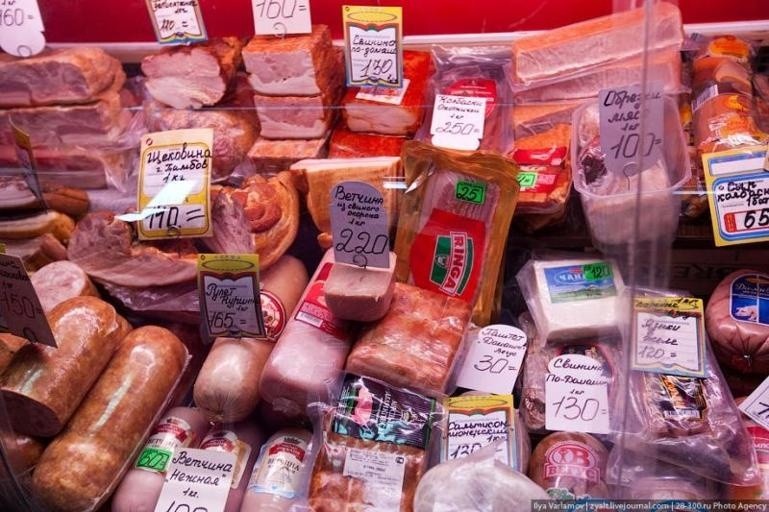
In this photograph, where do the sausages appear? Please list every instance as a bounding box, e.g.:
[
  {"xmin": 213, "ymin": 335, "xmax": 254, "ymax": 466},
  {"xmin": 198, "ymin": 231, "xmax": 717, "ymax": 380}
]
[
  {"xmin": 2, "ymin": 244, "xmax": 397, "ymax": 511},
  {"xmin": 705, "ymin": 263, "xmax": 768, "ymax": 372}
]
